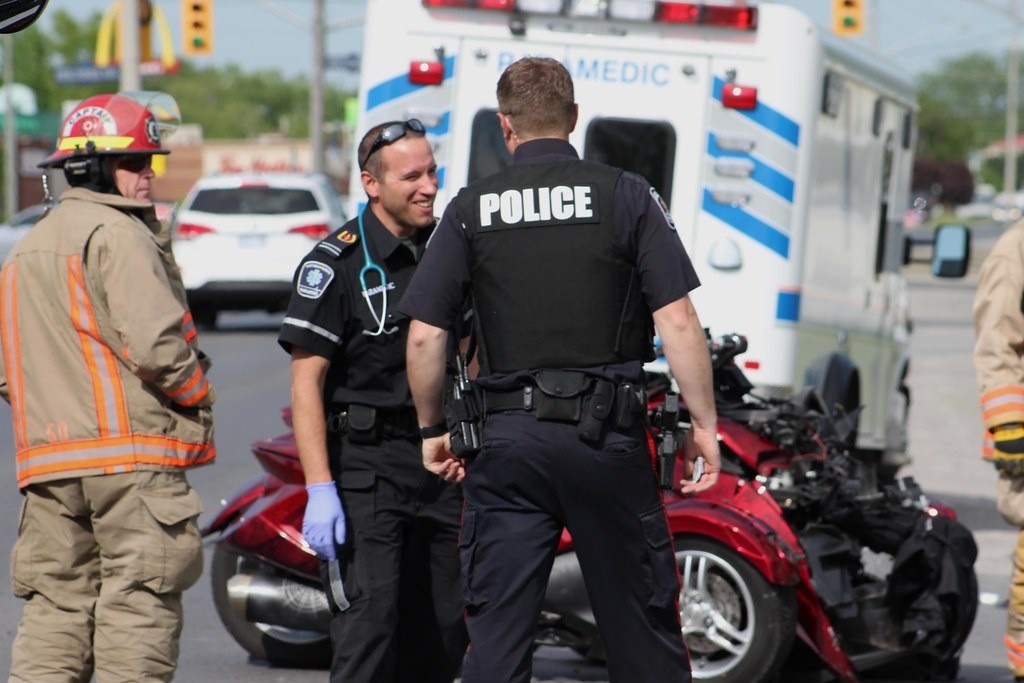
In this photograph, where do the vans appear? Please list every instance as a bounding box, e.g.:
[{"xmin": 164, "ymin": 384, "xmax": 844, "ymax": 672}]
[{"xmin": 352, "ymin": 0, "xmax": 971, "ymax": 487}]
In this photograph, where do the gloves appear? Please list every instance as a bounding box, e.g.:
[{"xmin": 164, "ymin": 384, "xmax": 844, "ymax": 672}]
[{"xmin": 302, "ymin": 481, "xmax": 347, "ymax": 563}]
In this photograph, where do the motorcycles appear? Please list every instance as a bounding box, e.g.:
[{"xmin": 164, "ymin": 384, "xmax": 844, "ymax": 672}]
[
  {"xmin": 675, "ymin": 329, "xmax": 979, "ymax": 667},
  {"xmin": 198, "ymin": 329, "xmax": 853, "ymax": 683}
]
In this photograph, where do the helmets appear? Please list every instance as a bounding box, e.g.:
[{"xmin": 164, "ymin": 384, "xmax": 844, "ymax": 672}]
[{"xmin": 36, "ymin": 94, "xmax": 172, "ymax": 169}]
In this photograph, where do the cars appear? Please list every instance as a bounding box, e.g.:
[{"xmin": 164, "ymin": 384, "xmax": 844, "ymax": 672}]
[{"xmin": 165, "ymin": 172, "xmax": 347, "ymax": 330}]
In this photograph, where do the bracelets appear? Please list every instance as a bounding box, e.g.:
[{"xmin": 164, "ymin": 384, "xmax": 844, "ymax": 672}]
[{"xmin": 416, "ymin": 420, "xmax": 450, "ymax": 440}]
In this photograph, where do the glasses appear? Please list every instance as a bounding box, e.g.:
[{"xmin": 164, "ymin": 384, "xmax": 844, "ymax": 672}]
[
  {"xmin": 359, "ymin": 120, "xmax": 426, "ymax": 172},
  {"xmin": 106, "ymin": 155, "xmax": 152, "ymax": 173}
]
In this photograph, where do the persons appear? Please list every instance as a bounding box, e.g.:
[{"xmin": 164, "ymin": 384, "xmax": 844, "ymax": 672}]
[
  {"xmin": 1, "ymin": 95, "xmax": 218, "ymax": 681},
  {"xmin": 970, "ymin": 211, "xmax": 1024, "ymax": 682},
  {"xmin": 275, "ymin": 117, "xmax": 483, "ymax": 682},
  {"xmin": 394, "ymin": 56, "xmax": 722, "ymax": 683}
]
